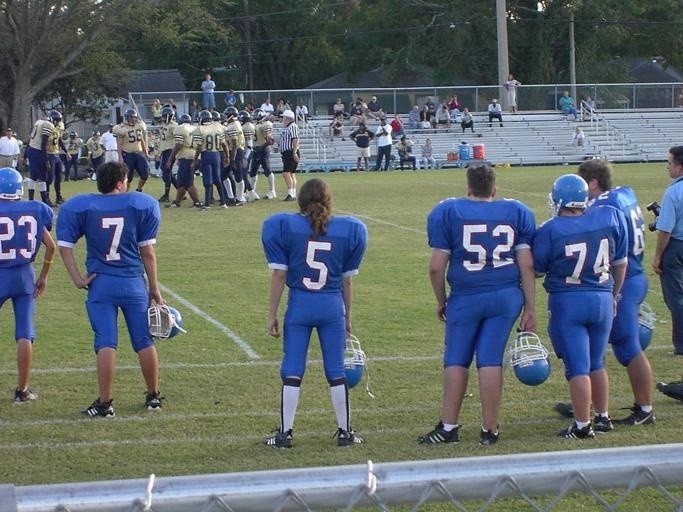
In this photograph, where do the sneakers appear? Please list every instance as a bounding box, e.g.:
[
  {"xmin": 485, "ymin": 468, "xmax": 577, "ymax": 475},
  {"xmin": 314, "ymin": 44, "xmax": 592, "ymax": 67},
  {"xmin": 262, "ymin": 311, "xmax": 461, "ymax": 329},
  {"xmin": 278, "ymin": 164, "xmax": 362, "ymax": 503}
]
[
  {"xmin": 655, "ymin": 380, "xmax": 683, "ymax": 403},
  {"xmin": 477, "ymin": 424, "xmax": 501, "ymax": 448},
  {"xmin": 336, "ymin": 427, "xmax": 367, "ymax": 448},
  {"xmin": 157, "ymin": 189, "xmax": 297, "ymax": 212},
  {"xmin": 142, "ymin": 391, "xmax": 164, "ymax": 413},
  {"xmin": 594, "ymin": 415, "xmax": 616, "ymax": 431},
  {"xmin": 611, "ymin": 404, "xmax": 657, "ymax": 427},
  {"xmin": 555, "ymin": 423, "xmax": 596, "ymax": 440},
  {"xmin": 553, "ymin": 402, "xmax": 594, "ymax": 419},
  {"xmin": 416, "ymin": 419, "xmax": 462, "ymax": 446},
  {"xmin": 13, "ymin": 386, "xmax": 38, "ymax": 406},
  {"xmin": 81, "ymin": 396, "xmax": 116, "ymax": 420},
  {"xmin": 263, "ymin": 427, "xmax": 294, "ymax": 449}
]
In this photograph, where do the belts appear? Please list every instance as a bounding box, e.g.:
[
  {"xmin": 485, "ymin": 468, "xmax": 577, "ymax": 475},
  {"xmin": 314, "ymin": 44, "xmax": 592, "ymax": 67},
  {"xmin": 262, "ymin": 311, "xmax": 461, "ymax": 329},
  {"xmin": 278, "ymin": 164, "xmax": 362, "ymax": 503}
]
[
  {"xmin": 0, "ymin": 154, "xmax": 9, "ymax": 157},
  {"xmin": 105, "ymin": 149, "xmax": 116, "ymax": 150}
]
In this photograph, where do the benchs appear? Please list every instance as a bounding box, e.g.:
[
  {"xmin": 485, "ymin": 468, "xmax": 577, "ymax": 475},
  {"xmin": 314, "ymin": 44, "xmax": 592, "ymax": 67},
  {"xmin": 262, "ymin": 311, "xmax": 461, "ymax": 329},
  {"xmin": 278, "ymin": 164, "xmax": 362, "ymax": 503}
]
[{"xmin": 269, "ymin": 106, "xmax": 682, "ymax": 172}]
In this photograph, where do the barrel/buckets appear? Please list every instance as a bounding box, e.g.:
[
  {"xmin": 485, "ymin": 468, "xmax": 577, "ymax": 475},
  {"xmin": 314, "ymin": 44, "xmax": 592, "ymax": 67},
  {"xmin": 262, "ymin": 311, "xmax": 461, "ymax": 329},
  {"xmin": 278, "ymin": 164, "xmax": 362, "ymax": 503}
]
[
  {"xmin": 473, "ymin": 144, "xmax": 484, "ymax": 158},
  {"xmin": 459, "ymin": 145, "xmax": 469, "ymax": 160}
]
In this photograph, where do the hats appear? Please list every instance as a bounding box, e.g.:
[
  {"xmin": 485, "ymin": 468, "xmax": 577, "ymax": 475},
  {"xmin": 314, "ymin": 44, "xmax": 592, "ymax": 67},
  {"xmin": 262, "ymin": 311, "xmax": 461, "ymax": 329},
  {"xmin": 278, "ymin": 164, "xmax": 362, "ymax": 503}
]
[{"xmin": 279, "ymin": 109, "xmax": 296, "ymax": 121}]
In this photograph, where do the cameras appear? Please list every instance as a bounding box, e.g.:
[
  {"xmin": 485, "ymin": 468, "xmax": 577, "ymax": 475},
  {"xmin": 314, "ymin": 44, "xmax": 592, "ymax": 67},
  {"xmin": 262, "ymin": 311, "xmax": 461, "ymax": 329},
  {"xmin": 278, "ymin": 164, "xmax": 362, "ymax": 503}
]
[{"xmin": 647, "ymin": 201, "xmax": 660, "ymax": 231}]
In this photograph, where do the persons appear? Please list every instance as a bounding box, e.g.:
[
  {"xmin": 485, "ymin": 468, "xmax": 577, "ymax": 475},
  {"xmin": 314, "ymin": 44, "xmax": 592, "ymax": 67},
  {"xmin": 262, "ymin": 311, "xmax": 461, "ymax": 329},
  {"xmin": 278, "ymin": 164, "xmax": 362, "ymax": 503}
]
[
  {"xmin": 199, "ymin": 73, "xmax": 216, "ymax": 111},
  {"xmin": 583, "ymin": 95, "xmax": 595, "ymax": 115},
  {"xmin": 529, "ymin": 172, "xmax": 628, "ymax": 439},
  {"xmin": 503, "ymin": 74, "xmax": 521, "ymax": 113},
  {"xmin": 55, "ymin": 160, "xmax": 167, "ymax": 418},
  {"xmin": 414, "ymin": 163, "xmax": 539, "ymax": 445},
  {"xmin": 554, "ymin": 158, "xmax": 656, "ymax": 426},
  {"xmin": 11, "ymin": 130, "xmax": 23, "ymax": 169},
  {"xmin": 0, "ymin": 128, "xmax": 20, "ymax": 170},
  {"xmin": 260, "ymin": 179, "xmax": 366, "ymax": 448},
  {"xmin": 0, "ymin": 166, "xmax": 56, "ymax": 405},
  {"xmin": 557, "ymin": 90, "xmax": 579, "ymax": 120},
  {"xmin": 572, "ymin": 127, "xmax": 584, "ymax": 146},
  {"xmin": 487, "ymin": 99, "xmax": 503, "ymax": 126},
  {"xmin": 654, "ymin": 144, "xmax": 682, "ymax": 402}
]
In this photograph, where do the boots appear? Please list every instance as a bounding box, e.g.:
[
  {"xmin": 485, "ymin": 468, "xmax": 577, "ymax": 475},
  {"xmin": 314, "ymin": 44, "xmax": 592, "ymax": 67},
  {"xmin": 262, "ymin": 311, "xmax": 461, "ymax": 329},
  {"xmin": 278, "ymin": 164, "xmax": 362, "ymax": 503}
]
[
  {"xmin": 28, "ymin": 189, "xmax": 35, "ymax": 200},
  {"xmin": 56, "ymin": 191, "xmax": 66, "ymax": 203},
  {"xmin": 39, "ymin": 190, "xmax": 56, "ymax": 208}
]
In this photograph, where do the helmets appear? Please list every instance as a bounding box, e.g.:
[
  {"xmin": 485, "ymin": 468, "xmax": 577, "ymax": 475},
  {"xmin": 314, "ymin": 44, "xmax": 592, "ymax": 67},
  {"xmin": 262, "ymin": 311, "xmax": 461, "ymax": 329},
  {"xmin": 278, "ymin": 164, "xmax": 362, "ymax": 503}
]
[
  {"xmin": 70, "ymin": 122, "xmax": 116, "ymax": 138},
  {"xmin": 506, "ymin": 331, "xmax": 552, "ymax": 386},
  {"xmin": 146, "ymin": 304, "xmax": 184, "ymax": 341},
  {"xmin": 161, "ymin": 106, "xmax": 174, "ymax": 118},
  {"xmin": 176, "ymin": 106, "xmax": 268, "ymax": 124},
  {"xmin": 343, "ymin": 331, "xmax": 366, "ymax": 391},
  {"xmin": 638, "ymin": 300, "xmax": 655, "ymax": 351},
  {"xmin": 0, "ymin": 167, "xmax": 25, "ymax": 202},
  {"xmin": 547, "ymin": 173, "xmax": 590, "ymax": 217},
  {"xmin": 123, "ymin": 109, "xmax": 139, "ymax": 121},
  {"xmin": 47, "ymin": 109, "xmax": 63, "ymax": 122}
]
